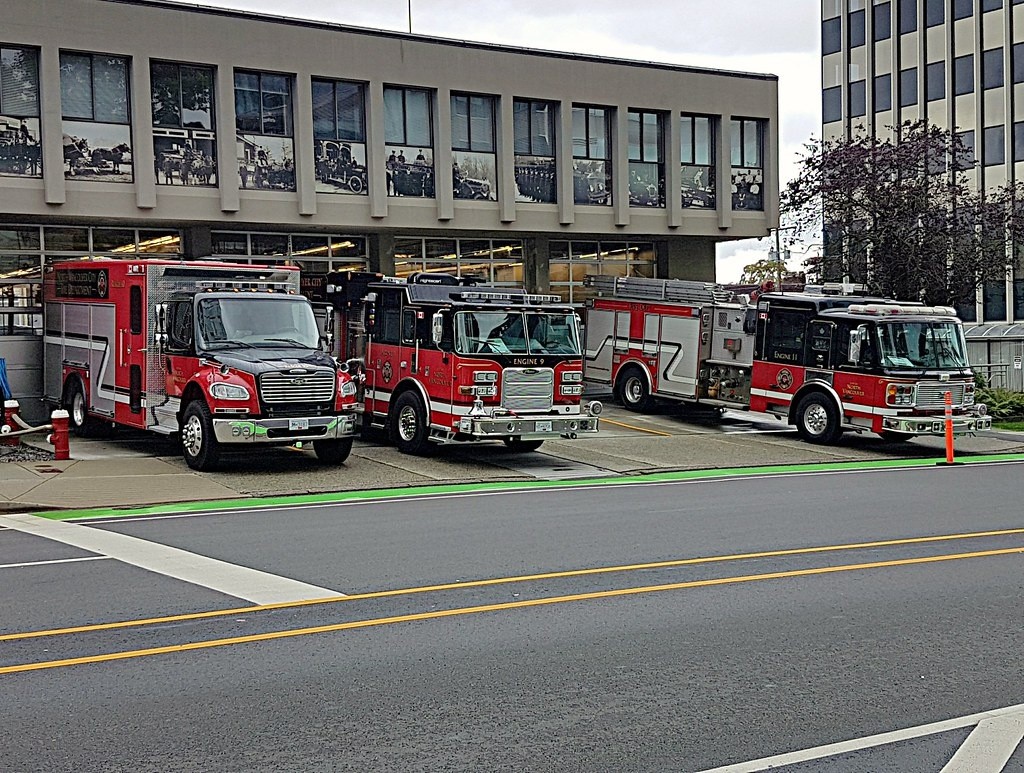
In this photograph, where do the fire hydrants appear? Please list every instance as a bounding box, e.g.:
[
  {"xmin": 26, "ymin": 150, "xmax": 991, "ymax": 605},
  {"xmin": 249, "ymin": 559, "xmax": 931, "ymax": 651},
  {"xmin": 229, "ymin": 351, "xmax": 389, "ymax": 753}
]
[
  {"xmin": 46, "ymin": 409, "xmax": 70, "ymax": 459},
  {"xmin": 1, "ymin": 400, "xmax": 21, "ymax": 447}
]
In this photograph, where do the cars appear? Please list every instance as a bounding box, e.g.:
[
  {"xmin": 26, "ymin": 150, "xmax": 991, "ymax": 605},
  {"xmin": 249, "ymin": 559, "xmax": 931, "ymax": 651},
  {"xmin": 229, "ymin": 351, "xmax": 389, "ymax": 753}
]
[{"xmin": 320, "ymin": 158, "xmax": 367, "ymax": 194}]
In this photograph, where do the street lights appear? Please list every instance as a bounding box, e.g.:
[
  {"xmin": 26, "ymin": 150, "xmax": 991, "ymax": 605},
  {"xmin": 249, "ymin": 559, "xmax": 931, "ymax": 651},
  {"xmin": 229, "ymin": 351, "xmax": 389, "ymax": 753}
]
[{"xmin": 768, "ymin": 226, "xmax": 796, "ymax": 292}]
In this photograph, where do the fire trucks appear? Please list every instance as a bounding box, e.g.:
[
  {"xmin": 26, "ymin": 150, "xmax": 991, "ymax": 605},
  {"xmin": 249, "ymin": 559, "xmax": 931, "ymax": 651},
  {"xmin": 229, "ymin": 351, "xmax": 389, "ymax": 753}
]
[
  {"xmin": 321, "ymin": 270, "xmax": 602, "ymax": 456},
  {"xmin": 581, "ymin": 275, "xmax": 992, "ymax": 446},
  {"xmin": 38, "ymin": 259, "xmax": 365, "ymax": 471}
]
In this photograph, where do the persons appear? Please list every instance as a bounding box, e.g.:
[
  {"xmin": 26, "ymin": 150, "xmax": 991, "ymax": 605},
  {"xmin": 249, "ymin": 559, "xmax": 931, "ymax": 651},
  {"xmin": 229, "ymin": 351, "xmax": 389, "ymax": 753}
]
[
  {"xmin": 732, "ymin": 170, "xmax": 762, "ymax": 211},
  {"xmin": 240, "ymin": 162, "xmax": 248, "ymax": 187},
  {"xmin": 179, "ymin": 159, "xmax": 189, "ymax": 186},
  {"xmin": 517, "ymin": 167, "xmax": 555, "ymax": 203},
  {"xmin": 389, "ymin": 149, "xmax": 405, "ymax": 164},
  {"xmin": 416, "ymin": 149, "xmax": 425, "ymax": 163},
  {"xmin": 183, "ymin": 140, "xmax": 192, "ymax": 157},
  {"xmin": 254, "ymin": 161, "xmax": 264, "ymax": 190},
  {"xmin": 352, "ymin": 156, "xmax": 357, "ymax": 168},
  {"xmin": 163, "ymin": 157, "xmax": 174, "ymax": 184},
  {"xmin": 190, "ymin": 153, "xmax": 214, "ymax": 185},
  {"xmin": 258, "ymin": 146, "xmax": 269, "ymax": 166},
  {"xmin": 154, "ymin": 156, "xmax": 161, "ymax": 184},
  {"xmin": 19, "ymin": 119, "xmax": 33, "ymax": 155},
  {"xmin": 453, "ymin": 162, "xmax": 462, "ymax": 194}
]
